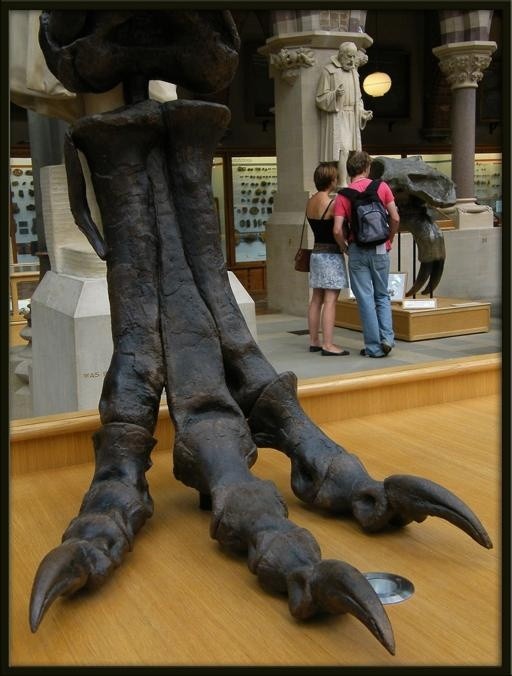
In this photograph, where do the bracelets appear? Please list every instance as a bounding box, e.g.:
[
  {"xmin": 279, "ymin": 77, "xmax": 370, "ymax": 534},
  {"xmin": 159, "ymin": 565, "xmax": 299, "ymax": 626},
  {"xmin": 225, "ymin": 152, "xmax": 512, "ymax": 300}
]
[{"xmin": 340, "ymin": 246, "xmax": 348, "ymax": 253}]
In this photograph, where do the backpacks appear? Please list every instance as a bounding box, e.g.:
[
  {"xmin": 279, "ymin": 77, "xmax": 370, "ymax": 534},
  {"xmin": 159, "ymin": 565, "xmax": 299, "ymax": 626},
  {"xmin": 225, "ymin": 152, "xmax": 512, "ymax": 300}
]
[{"xmin": 338, "ymin": 179, "xmax": 391, "ymax": 245}]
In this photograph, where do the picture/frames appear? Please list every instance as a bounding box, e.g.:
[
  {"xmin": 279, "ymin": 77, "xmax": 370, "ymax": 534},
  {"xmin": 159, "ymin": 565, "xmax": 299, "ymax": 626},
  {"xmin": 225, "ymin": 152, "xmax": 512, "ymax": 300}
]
[{"xmin": 387, "ymin": 271, "xmax": 408, "ymax": 304}]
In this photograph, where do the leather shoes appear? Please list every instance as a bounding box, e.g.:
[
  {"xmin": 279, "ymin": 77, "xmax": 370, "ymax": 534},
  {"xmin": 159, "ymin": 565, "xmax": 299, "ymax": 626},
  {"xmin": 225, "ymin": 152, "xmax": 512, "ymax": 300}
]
[{"xmin": 309, "ymin": 346, "xmax": 349, "ymax": 355}]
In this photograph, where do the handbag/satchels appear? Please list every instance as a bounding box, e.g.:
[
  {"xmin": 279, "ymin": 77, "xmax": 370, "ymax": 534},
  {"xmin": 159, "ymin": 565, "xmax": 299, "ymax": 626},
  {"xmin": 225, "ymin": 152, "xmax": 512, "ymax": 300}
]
[{"xmin": 294, "ymin": 248, "xmax": 312, "ymax": 272}]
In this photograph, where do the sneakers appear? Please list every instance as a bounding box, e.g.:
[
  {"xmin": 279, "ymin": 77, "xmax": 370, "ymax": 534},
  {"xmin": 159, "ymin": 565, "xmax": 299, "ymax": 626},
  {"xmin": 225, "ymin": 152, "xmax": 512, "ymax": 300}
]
[{"xmin": 361, "ymin": 343, "xmax": 391, "ymax": 357}]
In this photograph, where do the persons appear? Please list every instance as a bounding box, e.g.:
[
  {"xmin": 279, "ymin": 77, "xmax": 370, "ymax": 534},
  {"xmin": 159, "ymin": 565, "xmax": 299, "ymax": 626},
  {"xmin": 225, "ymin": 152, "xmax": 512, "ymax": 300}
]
[
  {"xmin": 234, "ymin": 229, "xmax": 257, "ymax": 262},
  {"xmin": 315, "ymin": 40, "xmax": 375, "ymax": 187},
  {"xmin": 244, "ymin": 232, "xmax": 266, "ymax": 259},
  {"xmin": 331, "ymin": 149, "xmax": 401, "ymax": 358},
  {"xmin": 305, "ymin": 162, "xmax": 351, "ymax": 358}
]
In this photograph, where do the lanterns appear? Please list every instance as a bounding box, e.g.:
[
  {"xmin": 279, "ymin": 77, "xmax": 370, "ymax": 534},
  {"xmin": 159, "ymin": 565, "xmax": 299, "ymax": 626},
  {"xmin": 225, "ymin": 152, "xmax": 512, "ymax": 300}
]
[{"xmin": 361, "ymin": 71, "xmax": 393, "ymax": 99}]
